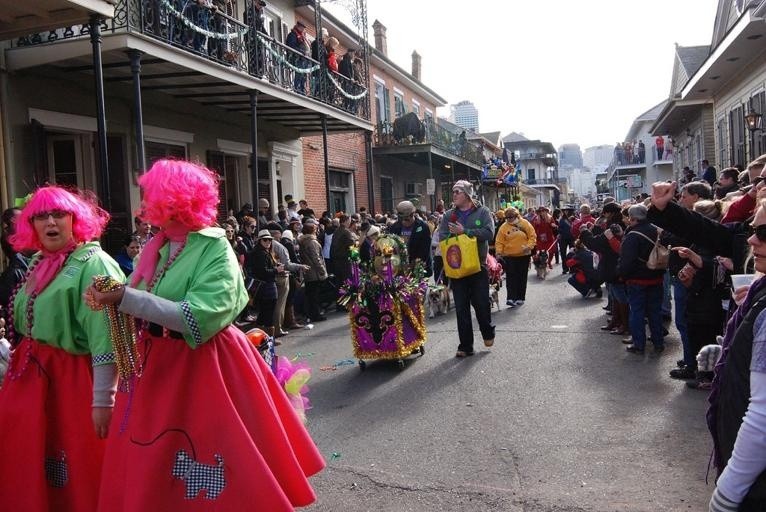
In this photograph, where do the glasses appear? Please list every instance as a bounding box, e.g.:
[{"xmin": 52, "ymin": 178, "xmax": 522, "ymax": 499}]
[
  {"xmin": 452, "ymin": 189, "xmax": 464, "ymax": 194},
  {"xmin": 32, "ymin": 210, "xmax": 72, "ymax": 224},
  {"xmin": 745, "ymin": 223, "xmax": 766, "ymax": 242},
  {"xmin": 226, "ymin": 205, "xmax": 272, "ymax": 242}
]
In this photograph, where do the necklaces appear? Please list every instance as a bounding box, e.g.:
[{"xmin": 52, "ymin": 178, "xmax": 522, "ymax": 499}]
[{"xmin": 89, "ymin": 274, "xmax": 142, "ymax": 381}]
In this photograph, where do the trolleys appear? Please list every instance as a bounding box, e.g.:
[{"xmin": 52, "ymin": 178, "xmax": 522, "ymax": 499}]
[{"xmin": 341, "ymin": 262, "xmax": 428, "ymax": 370}]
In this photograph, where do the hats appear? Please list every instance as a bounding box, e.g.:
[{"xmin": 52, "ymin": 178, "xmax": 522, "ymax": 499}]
[
  {"xmin": 452, "ymin": 180, "xmax": 473, "ymax": 200},
  {"xmin": 536, "ymin": 206, "xmax": 550, "ymax": 214},
  {"xmin": 282, "ymin": 229, "xmax": 293, "ymax": 241},
  {"xmin": 396, "ymin": 201, "xmax": 415, "ymax": 217},
  {"xmin": 290, "ymin": 216, "xmax": 301, "ymax": 224},
  {"xmin": 561, "ymin": 204, "xmax": 576, "ymax": 211}
]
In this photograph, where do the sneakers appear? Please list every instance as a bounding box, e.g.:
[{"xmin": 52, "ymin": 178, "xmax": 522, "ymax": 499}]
[
  {"xmin": 455, "ymin": 350, "xmax": 474, "ymax": 357},
  {"xmin": 505, "ymin": 299, "xmax": 517, "ymax": 305},
  {"xmin": 484, "ymin": 338, "xmax": 494, "ymax": 346},
  {"xmin": 584, "ymin": 288, "xmax": 592, "ymax": 299},
  {"xmin": 517, "ymin": 299, "xmax": 525, "ymax": 305},
  {"xmin": 232, "ymin": 311, "xmax": 327, "ymax": 346}
]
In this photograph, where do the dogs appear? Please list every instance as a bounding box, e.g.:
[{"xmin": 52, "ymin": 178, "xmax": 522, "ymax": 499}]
[
  {"xmin": 488, "ymin": 283, "xmax": 501, "ymax": 313},
  {"xmin": 427, "ymin": 284, "xmax": 451, "ymax": 319},
  {"xmin": 534, "ymin": 248, "xmax": 549, "ymax": 280}
]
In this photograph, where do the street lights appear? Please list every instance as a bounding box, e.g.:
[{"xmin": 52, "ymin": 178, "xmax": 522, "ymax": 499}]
[{"xmin": 743, "ymin": 96, "xmax": 763, "ymax": 164}]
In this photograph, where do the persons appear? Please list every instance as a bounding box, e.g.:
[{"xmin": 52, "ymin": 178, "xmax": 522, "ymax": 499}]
[
  {"xmin": 83, "ymin": 158, "xmax": 324, "ymax": 511},
  {"xmin": 144, "ymin": 0, "xmax": 363, "ymax": 115},
  {"xmin": 439, "ymin": 179, "xmax": 496, "ymax": 357},
  {"xmin": 1, "ymin": 184, "xmax": 127, "ymax": 511},
  {"xmin": 115, "ymin": 192, "xmax": 447, "ymax": 347},
  {"xmin": 565, "ymin": 152, "xmax": 766, "ymax": 510},
  {"xmin": 485, "ymin": 206, "xmax": 603, "ymax": 306}
]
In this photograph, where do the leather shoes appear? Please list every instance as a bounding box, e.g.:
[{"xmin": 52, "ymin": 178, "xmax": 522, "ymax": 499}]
[{"xmin": 601, "ymin": 293, "xmax": 714, "ymax": 390}]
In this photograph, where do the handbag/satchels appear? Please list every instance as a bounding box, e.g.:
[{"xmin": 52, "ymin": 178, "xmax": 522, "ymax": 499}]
[
  {"xmin": 438, "ymin": 233, "xmax": 481, "ymax": 280},
  {"xmin": 290, "ymin": 274, "xmax": 306, "ymax": 298},
  {"xmin": 243, "ymin": 275, "xmax": 267, "ymax": 300}
]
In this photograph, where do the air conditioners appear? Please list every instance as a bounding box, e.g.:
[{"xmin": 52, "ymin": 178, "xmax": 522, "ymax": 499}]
[{"xmin": 405, "ymin": 183, "xmax": 423, "ymax": 196}]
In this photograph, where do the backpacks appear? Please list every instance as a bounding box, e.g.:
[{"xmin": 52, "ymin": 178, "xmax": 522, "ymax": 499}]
[{"xmin": 626, "ymin": 230, "xmax": 672, "ymax": 270}]
